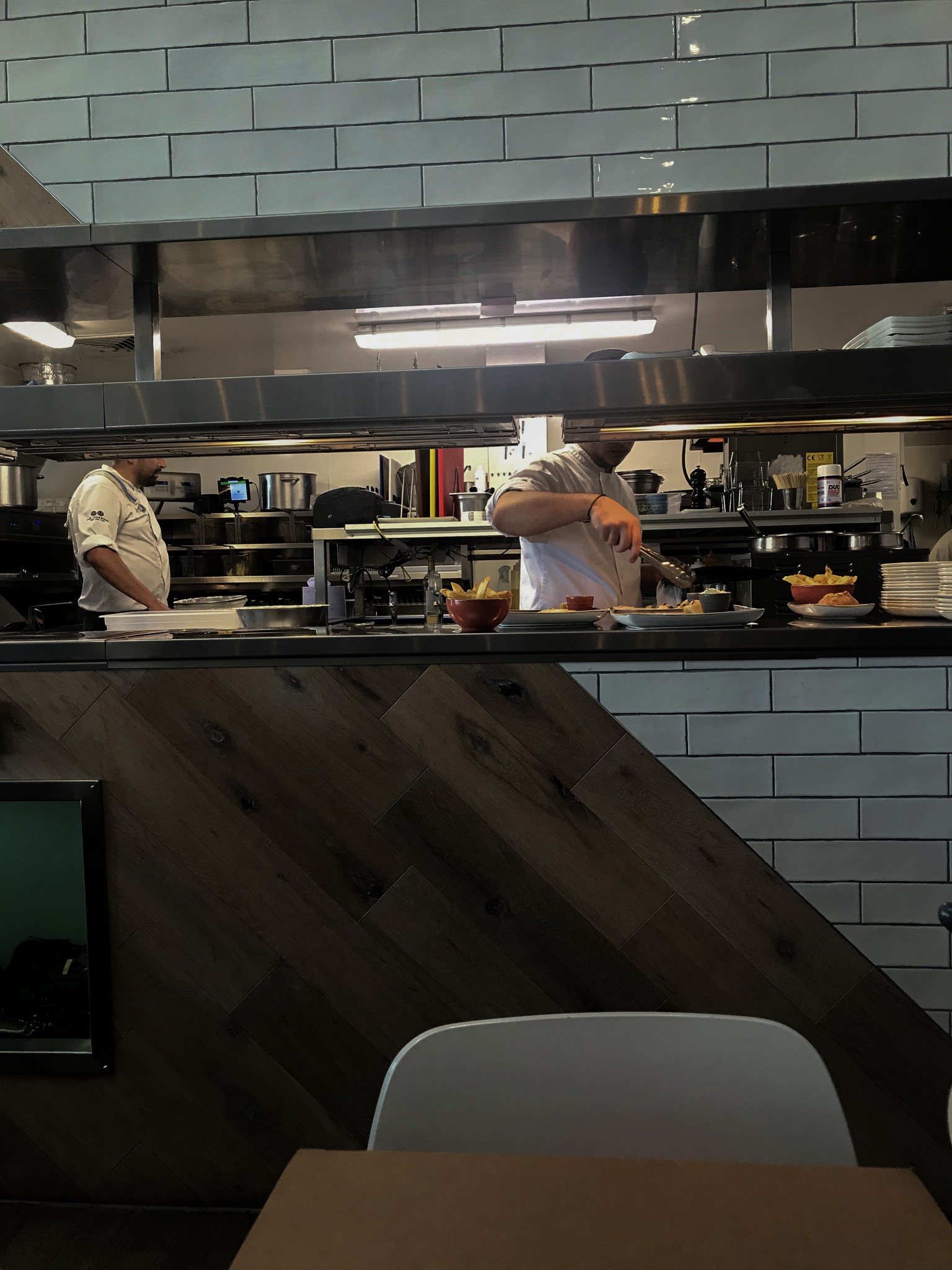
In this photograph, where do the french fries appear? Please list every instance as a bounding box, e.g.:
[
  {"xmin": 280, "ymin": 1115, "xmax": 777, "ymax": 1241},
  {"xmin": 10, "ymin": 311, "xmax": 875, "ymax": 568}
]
[
  {"xmin": 439, "ymin": 575, "xmax": 513, "ymax": 599},
  {"xmin": 782, "ymin": 566, "xmax": 857, "ymax": 585}
]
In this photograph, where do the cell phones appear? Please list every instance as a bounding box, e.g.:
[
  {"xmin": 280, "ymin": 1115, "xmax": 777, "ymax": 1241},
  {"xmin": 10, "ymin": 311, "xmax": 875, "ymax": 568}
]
[{"xmin": 217, "ymin": 479, "xmax": 251, "ymax": 502}]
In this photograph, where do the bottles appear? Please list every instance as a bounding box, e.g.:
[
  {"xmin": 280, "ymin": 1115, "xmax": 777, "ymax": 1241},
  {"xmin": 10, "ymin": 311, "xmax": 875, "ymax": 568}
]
[
  {"xmin": 474, "ymin": 465, "xmax": 488, "ymax": 492},
  {"xmin": 423, "ymin": 556, "xmax": 443, "ymax": 630},
  {"xmin": 817, "ymin": 464, "xmax": 843, "ymax": 508},
  {"xmin": 510, "ymin": 565, "xmax": 520, "ymax": 610}
]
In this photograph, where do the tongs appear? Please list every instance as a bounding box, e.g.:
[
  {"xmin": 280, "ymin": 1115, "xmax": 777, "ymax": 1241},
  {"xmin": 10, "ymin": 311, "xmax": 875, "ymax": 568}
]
[{"xmin": 628, "ymin": 542, "xmax": 696, "ymax": 589}]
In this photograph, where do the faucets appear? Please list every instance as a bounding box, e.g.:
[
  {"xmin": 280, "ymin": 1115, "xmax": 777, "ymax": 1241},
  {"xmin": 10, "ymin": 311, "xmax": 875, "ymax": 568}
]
[
  {"xmin": 387, "ymin": 590, "xmax": 398, "ymax": 626},
  {"xmin": 431, "ymin": 590, "xmax": 445, "ymax": 625}
]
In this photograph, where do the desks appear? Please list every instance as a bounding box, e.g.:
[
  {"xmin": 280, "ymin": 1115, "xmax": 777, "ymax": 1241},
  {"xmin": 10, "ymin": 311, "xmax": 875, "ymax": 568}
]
[{"xmin": 225, "ymin": 1153, "xmax": 952, "ymax": 1270}]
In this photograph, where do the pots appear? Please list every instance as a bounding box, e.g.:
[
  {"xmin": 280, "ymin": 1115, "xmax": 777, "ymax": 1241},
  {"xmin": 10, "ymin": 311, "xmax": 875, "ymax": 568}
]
[
  {"xmin": 0, "ymin": 463, "xmax": 44, "ymax": 510},
  {"xmin": 257, "ymin": 472, "xmax": 317, "ymax": 512}
]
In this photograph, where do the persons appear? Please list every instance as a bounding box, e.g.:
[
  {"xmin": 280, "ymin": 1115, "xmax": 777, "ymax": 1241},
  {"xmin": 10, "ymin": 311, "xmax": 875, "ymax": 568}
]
[
  {"xmin": 64, "ymin": 458, "xmax": 174, "ymax": 631},
  {"xmin": 484, "ymin": 441, "xmax": 643, "ymax": 610}
]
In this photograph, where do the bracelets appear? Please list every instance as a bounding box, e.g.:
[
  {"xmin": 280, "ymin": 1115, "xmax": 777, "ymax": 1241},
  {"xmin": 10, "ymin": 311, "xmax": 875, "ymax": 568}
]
[{"xmin": 587, "ymin": 494, "xmax": 607, "ymax": 519}]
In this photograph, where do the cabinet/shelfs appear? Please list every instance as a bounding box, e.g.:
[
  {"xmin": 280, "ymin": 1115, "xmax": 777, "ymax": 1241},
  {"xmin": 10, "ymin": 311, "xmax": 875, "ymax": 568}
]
[{"xmin": 157, "ymin": 510, "xmax": 474, "ymax": 591}]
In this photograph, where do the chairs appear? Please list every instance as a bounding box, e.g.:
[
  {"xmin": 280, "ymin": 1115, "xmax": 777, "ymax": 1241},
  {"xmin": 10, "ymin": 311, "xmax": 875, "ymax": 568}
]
[{"xmin": 363, "ymin": 1013, "xmax": 858, "ymax": 1170}]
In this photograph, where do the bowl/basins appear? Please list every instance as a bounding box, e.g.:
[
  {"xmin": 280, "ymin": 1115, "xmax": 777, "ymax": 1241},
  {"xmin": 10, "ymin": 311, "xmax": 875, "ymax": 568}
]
[
  {"xmin": 230, "ymin": 605, "xmax": 331, "ymax": 628},
  {"xmin": 635, "ymin": 494, "xmax": 668, "ymax": 515},
  {"xmin": 615, "ymin": 469, "xmax": 664, "ymax": 494},
  {"xmin": 446, "ymin": 598, "xmax": 511, "ymax": 630},
  {"xmin": 790, "ymin": 584, "xmax": 855, "ymax": 603}
]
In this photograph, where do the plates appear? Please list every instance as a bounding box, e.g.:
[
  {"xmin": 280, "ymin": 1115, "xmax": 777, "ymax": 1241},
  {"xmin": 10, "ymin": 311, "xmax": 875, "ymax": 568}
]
[
  {"xmin": 842, "ymin": 314, "xmax": 952, "ymax": 349},
  {"xmin": 934, "ymin": 563, "xmax": 952, "ymax": 620},
  {"xmin": 880, "ymin": 561, "xmax": 952, "ymax": 616},
  {"xmin": 611, "ymin": 605, "xmax": 764, "ymax": 628},
  {"xmin": 499, "ymin": 609, "xmax": 609, "ymax": 626},
  {"xmin": 787, "ymin": 602, "xmax": 875, "ymax": 619}
]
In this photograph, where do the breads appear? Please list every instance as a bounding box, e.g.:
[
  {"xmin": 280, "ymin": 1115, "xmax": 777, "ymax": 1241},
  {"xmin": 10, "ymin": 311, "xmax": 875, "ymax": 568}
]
[{"xmin": 817, "ymin": 591, "xmax": 860, "ymax": 605}]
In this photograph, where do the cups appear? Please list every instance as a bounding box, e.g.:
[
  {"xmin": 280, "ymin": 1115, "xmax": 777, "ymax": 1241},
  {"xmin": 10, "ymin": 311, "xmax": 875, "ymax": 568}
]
[
  {"xmin": 566, "ymin": 595, "xmax": 594, "ymax": 611},
  {"xmin": 686, "ymin": 593, "xmax": 699, "ymax": 604},
  {"xmin": 779, "ymin": 487, "xmax": 804, "ymax": 510},
  {"xmin": 699, "ymin": 593, "xmax": 731, "ymax": 613}
]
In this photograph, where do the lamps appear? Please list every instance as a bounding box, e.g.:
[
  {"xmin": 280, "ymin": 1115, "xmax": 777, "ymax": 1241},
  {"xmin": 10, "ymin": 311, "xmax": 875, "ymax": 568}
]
[
  {"xmin": 353, "ymin": 307, "xmax": 658, "ymax": 352},
  {"xmin": 3, "ymin": 319, "xmax": 78, "ymax": 350}
]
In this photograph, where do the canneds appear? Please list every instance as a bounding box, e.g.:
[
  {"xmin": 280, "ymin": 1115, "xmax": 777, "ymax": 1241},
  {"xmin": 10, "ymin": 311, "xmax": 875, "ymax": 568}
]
[{"xmin": 817, "ymin": 464, "xmax": 842, "ymax": 508}]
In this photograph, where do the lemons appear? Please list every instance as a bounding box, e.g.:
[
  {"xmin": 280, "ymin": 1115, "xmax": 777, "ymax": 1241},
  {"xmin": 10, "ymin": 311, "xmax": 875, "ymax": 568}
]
[{"xmin": 683, "ymin": 600, "xmax": 705, "ymax": 614}]
